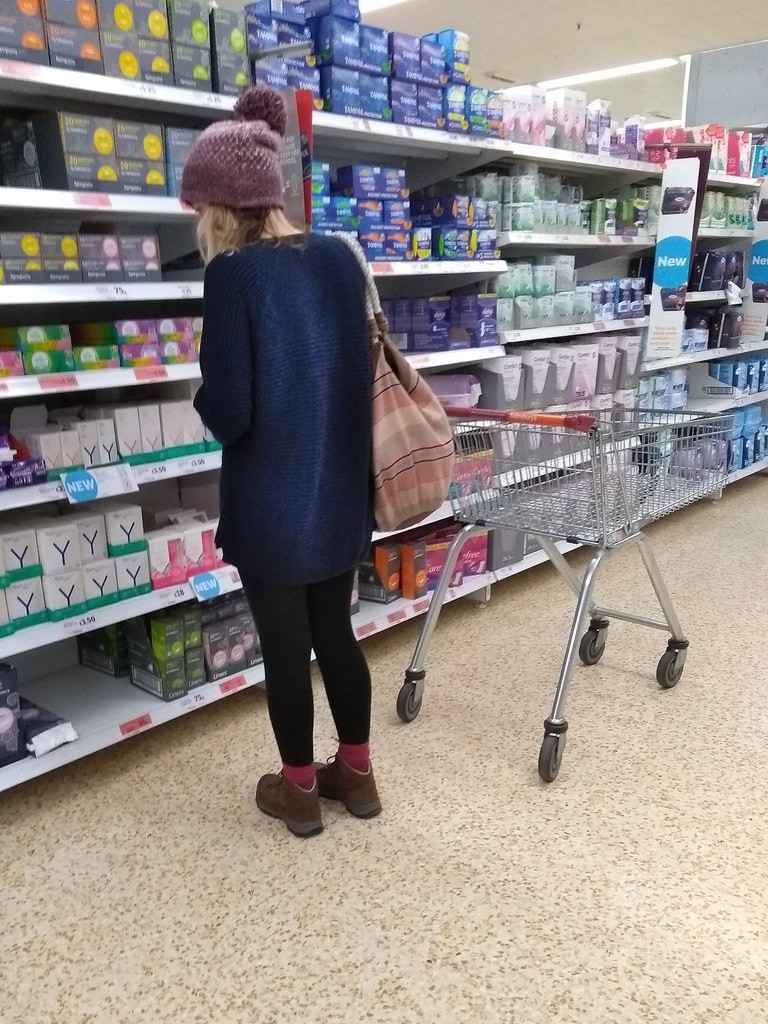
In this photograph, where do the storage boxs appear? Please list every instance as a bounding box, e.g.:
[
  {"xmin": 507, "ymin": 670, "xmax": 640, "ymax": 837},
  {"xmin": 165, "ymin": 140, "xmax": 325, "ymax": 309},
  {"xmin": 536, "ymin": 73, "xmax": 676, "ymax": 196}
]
[{"xmin": 1, "ymin": 0, "xmax": 500, "ymax": 702}]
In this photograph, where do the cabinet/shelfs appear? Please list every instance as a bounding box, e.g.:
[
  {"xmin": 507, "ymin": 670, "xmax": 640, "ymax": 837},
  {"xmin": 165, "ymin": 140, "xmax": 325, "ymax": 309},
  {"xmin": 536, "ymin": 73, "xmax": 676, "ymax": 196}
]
[{"xmin": 1, "ymin": 60, "xmax": 768, "ymax": 796}]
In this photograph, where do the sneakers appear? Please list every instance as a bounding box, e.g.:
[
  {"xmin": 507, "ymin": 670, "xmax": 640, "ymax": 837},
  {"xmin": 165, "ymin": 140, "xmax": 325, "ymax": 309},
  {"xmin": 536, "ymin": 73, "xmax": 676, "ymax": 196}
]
[
  {"xmin": 256, "ymin": 765, "xmax": 325, "ymax": 838},
  {"xmin": 312, "ymin": 749, "xmax": 383, "ymax": 820}
]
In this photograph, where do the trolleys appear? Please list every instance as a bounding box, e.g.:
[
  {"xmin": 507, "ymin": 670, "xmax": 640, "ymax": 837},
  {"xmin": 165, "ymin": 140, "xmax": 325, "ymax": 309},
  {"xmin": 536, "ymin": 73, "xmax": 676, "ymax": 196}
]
[{"xmin": 395, "ymin": 405, "xmax": 736, "ymax": 782}]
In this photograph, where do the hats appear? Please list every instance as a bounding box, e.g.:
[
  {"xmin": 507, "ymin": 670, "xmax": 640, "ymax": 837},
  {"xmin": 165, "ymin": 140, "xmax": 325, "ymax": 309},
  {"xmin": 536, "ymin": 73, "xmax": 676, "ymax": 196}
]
[{"xmin": 179, "ymin": 85, "xmax": 287, "ymax": 208}]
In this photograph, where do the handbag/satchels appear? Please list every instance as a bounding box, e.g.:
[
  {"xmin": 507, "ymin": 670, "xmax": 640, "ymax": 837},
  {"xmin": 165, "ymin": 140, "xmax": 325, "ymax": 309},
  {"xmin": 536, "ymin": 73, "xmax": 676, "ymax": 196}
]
[{"xmin": 332, "ymin": 230, "xmax": 455, "ymax": 533}]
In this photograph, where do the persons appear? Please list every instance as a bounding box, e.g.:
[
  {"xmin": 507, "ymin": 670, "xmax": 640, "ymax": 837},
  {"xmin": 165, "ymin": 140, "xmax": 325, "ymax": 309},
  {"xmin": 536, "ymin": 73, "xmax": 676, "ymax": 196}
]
[{"xmin": 182, "ymin": 84, "xmax": 384, "ymax": 837}]
